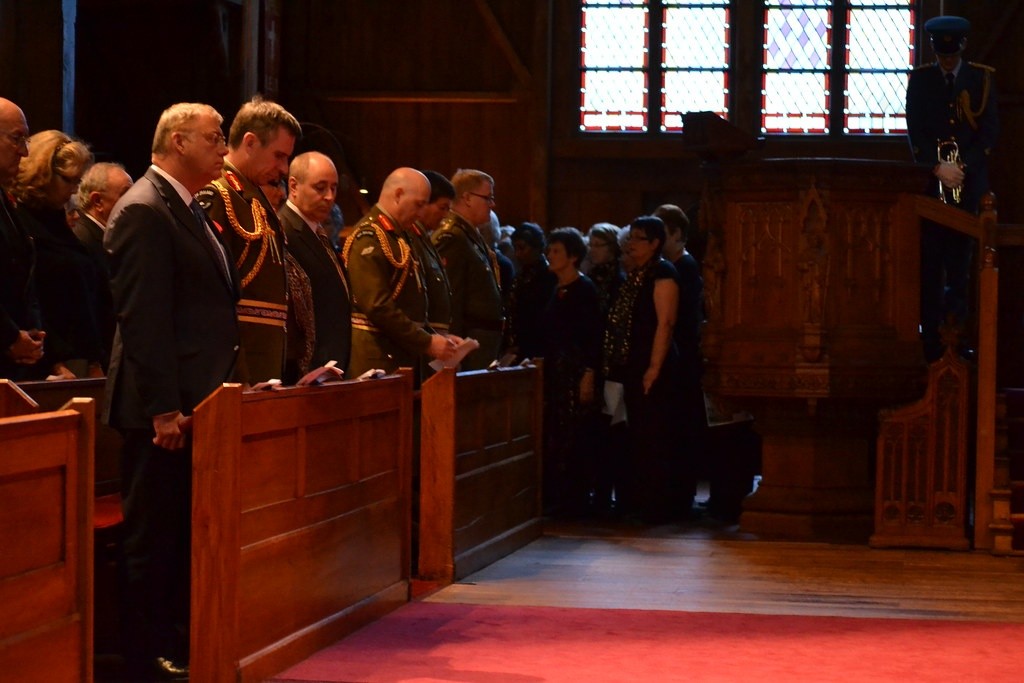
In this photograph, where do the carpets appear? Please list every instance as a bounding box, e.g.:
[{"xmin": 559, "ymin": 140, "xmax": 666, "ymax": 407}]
[{"xmin": 278, "ymin": 602, "xmax": 1024, "ymax": 683}]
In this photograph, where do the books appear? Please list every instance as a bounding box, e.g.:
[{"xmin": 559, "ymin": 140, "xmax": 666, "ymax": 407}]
[{"xmin": 429, "ymin": 338, "xmax": 478, "ymax": 372}]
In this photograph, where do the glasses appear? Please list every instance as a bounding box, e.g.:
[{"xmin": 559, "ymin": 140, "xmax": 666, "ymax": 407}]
[
  {"xmin": 626, "ymin": 233, "xmax": 647, "ymax": 242},
  {"xmin": 176, "ymin": 128, "xmax": 227, "ymax": 148},
  {"xmin": 0, "ymin": 130, "xmax": 31, "ymax": 150},
  {"xmin": 52, "ymin": 169, "xmax": 81, "ymax": 186},
  {"xmin": 585, "ymin": 242, "xmax": 608, "ymax": 251},
  {"xmin": 461, "ymin": 192, "xmax": 495, "ymax": 204}
]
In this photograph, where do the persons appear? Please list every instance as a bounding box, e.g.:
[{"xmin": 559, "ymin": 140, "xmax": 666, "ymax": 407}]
[
  {"xmin": 480, "ymin": 204, "xmax": 726, "ymax": 516},
  {"xmin": 906, "ymin": 17, "xmax": 999, "ymax": 356},
  {"xmin": 432, "ymin": 171, "xmax": 531, "ymax": 370},
  {"xmin": 0, "ymin": 96, "xmax": 131, "ymax": 384},
  {"xmin": 345, "ymin": 166, "xmax": 458, "ymax": 386},
  {"xmin": 103, "ymin": 102, "xmax": 353, "ymax": 680}
]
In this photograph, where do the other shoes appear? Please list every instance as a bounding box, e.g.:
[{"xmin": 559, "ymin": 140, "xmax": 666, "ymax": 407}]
[
  {"xmin": 152, "ymin": 653, "xmax": 190, "ymax": 678},
  {"xmin": 679, "ymin": 499, "xmax": 705, "ymax": 521},
  {"xmin": 955, "ymin": 339, "xmax": 979, "ymax": 366}
]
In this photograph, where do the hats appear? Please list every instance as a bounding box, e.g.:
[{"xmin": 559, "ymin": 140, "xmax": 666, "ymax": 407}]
[{"xmin": 924, "ymin": 16, "xmax": 969, "ymax": 54}]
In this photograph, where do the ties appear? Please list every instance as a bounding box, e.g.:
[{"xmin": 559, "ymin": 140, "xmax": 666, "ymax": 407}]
[
  {"xmin": 944, "ymin": 74, "xmax": 954, "ymax": 103},
  {"xmin": 423, "ymin": 233, "xmax": 444, "ymax": 274},
  {"xmin": 0, "ymin": 187, "xmax": 33, "ymax": 255},
  {"xmin": 316, "ymin": 225, "xmax": 350, "ymax": 300},
  {"xmin": 189, "ymin": 198, "xmax": 235, "ymax": 303},
  {"xmin": 402, "ymin": 229, "xmax": 423, "ymax": 289},
  {"xmin": 479, "ymin": 233, "xmax": 501, "ymax": 292}
]
